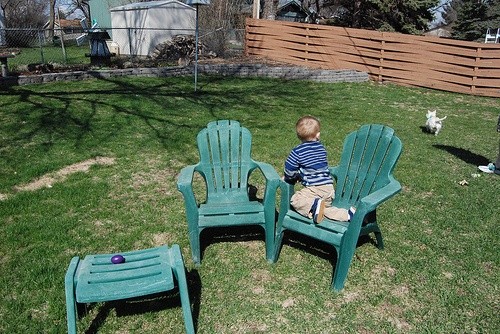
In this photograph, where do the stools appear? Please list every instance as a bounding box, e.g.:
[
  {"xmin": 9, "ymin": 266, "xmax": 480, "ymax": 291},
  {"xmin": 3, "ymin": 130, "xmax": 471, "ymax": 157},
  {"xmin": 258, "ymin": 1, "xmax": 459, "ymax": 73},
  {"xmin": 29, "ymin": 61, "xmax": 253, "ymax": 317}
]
[{"xmin": 64, "ymin": 244, "xmax": 195, "ymax": 334}]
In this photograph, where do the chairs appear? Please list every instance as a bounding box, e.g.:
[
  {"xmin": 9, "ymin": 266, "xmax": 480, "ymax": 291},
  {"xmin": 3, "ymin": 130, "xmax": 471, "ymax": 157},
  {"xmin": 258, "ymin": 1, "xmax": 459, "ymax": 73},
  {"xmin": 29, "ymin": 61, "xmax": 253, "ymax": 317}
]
[
  {"xmin": 176, "ymin": 119, "xmax": 281, "ymax": 267},
  {"xmin": 269, "ymin": 124, "xmax": 403, "ymax": 293}
]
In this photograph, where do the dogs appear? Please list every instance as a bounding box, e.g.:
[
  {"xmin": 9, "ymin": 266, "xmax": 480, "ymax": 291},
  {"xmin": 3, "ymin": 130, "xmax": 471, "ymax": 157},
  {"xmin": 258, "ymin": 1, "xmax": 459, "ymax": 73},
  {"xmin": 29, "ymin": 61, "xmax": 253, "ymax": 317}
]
[{"xmin": 425, "ymin": 109, "xmax": 447, "ymax": 137}]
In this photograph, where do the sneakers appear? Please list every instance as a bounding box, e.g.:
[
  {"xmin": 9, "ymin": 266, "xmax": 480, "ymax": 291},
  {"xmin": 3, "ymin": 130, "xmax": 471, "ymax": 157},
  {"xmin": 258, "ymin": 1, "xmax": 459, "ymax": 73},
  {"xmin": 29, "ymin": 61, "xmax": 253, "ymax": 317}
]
[
  {"xmin": 348, "ymin": 207, "xmax": 356, "ymax": 222},
  {"xmin": 310, "ymin": 198, "xmax": 325, "ymax": 223}
]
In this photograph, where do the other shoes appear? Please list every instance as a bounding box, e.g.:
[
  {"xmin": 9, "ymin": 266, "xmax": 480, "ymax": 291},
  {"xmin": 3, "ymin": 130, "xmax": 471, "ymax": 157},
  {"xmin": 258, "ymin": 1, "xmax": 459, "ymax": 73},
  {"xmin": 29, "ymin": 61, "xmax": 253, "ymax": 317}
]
[{"xmin": 478, "ymin": 163, "xmax": 495, "ymax": 174}]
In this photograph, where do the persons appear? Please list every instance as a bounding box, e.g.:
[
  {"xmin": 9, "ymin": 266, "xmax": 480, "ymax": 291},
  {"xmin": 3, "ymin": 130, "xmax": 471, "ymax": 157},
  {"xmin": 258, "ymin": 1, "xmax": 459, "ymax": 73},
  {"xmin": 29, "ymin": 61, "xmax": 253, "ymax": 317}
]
[
  {"xmin": 478, "ymin": 115, "xmax": 500, "ymax": 174},
  {"xmin": 281, "ymin": 116, "xmax": 357, "ymax": 225}
]
